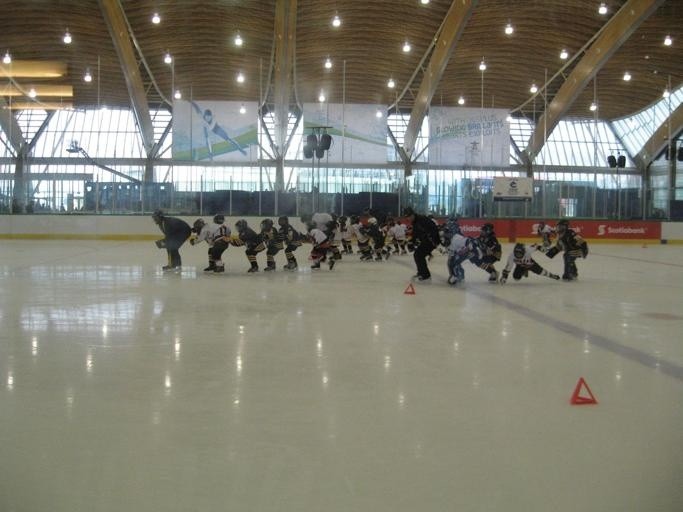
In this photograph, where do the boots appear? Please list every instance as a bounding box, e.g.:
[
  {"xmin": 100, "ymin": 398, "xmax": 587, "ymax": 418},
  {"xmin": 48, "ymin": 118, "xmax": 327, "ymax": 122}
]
[
  {"xmin": 524, "ymin": 270, "xmax": 528, "ymax": 278},
  {"xmin": 311, "ymin": 246, "xmax": 415, "ymax": 270},
  {"xmin": 489, "ymin": 269, "xmax": 500, "ymax": 281},
  {"xmin": 448, "ymin": 268, "xmax": 464, "ymax": 284},
  {"xmin": 414, "ymin": 272, "xmax": 431, "ymax": 280},
  {"xmin": 163, "ymin": 262, "xmax": 181, "ymax": 270},
  {"xmin": 550, "ymin": 272, "xmax": 578, "ymax": 280},
  {"xmin": 204, "ymin": 261, "xmax": 224, "ymax": 272},
  {"xmin": 248, "ymin": 262, "xmax": 258, "ymax": 272},
  {"xmin": 265, "ymin": 261, "xmax": 275, "ymax": 270},
  {"xmin": 284, "ymin": 256, "xmax": 297, "ymax": 269}
]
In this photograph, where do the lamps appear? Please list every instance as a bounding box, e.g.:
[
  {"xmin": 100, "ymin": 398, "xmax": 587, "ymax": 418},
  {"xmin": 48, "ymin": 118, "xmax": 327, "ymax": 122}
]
[
  {"xmin": 374, "ymin": 35, "xmax": 411, "ymax": 120},
  {"xmin": 530, "ymin": 0, "xmax": 674, "ymax": 112},
  {"xmin": 318, "ymin": 0, "xmax": 341, "ymax": 103},
  {"xmin": 457, "ymin": 18, "xmax": 514, "ymax": 105},
  {"xmin": 3, "ymin": 27, "xmax": 93, "ymax": 99},
  {"xmin": 151, "ymin": 12, "xmax": 182, "ymax": 99},
  {"xmin": 233, "ymin": 29, "xmax": 247, "ymax": 115}
]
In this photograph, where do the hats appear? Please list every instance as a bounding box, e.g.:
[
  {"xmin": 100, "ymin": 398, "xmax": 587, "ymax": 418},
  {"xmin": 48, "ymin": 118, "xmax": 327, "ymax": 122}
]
[{"xmin": 404, "ymin": 208, "xmax": 413, "ymax": 217}]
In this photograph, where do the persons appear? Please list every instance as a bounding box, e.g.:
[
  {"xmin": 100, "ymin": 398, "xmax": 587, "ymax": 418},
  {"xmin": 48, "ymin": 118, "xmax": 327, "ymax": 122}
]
[
  {"xmin": 0, "ymin": 196, "xmax": 87, "ymax": 214},
  {"xmin": 188, "ymin": 99, "xmax": 248, "ymax": 161}
]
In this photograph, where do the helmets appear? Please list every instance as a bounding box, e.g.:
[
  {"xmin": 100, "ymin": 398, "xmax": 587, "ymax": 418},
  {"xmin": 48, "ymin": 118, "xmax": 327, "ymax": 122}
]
[
  {"xmin": 152, "ymin": 211, "xmax": 163, "ymax": 224},
  {"xmin": 260, "ymin": 219, "xmax": 273, "ymax": 230},
  {"xmin": 213, "ymin": 214, "xmax": 224, "ymax": 224},
  {"xmin": 326, "ymin": 215, "xmax": 377, "ymax": 230},
  {"xmin": 556, "ymin": 220, "xmax": 568, "ymax": 233},
  {"xmin": 279, "ymin": 216, "xmax": 288, "ymax": 226},
  {"xmin": 480, "ymin": 224, "xmax": 493, "ymax": 237},
  {"xmin": 514, "ymin": 244, "xmax": 524, "ymax": 258},
  {"xmin": 235, "ymin": 219, "xmax": 247, "ymax": 232},
  {"xmin": 439, "ymin": 228, "xmax": 452, "ymax": 246},
  {"xmin": 308, "ymin": 221, "xmax": 316, "ymax": 230},
  {"xmin": 194, "ymin": 219, "xmax": 204, "ymax": 231}
]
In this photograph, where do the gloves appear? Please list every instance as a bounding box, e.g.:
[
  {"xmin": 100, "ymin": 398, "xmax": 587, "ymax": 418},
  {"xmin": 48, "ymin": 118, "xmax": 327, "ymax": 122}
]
[
  {"xmin": 501, "ymin": 270, "xmax": 510, "ymax": 285},
  {"xmin": 190, "ymin": 238, "xmax": 194, "ymax": 246},
  {"xmin": 582, "ymin": 242, "xmax": 588, "ymax": 258}
]
